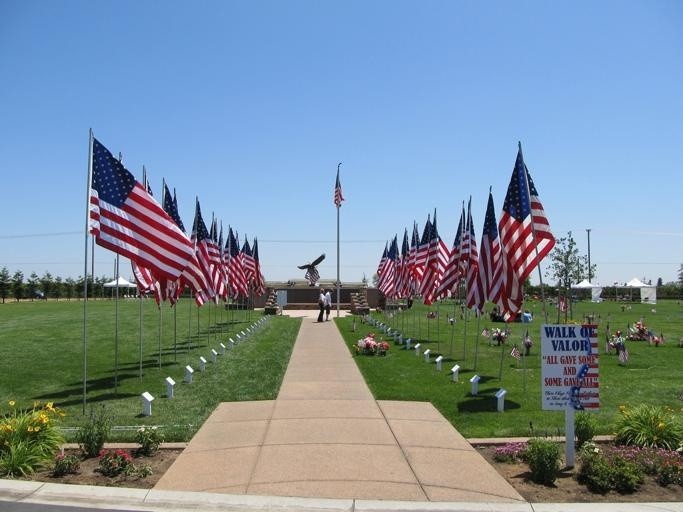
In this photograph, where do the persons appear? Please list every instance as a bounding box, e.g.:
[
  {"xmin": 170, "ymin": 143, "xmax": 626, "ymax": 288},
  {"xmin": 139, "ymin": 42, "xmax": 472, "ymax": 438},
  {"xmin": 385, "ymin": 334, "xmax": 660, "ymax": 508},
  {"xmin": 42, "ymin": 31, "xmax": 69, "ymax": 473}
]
[
  {"xmin": 324, "ymin": 288, "xmax": 334, "ymax": 321},
  {"xmin": 490, "ymin": 307, "xmax": 533, "ymax": 323},
  {"xmin": 560, "ymin": 294, "xmax": 565, "ymax": 311},
  {"xmin": 317, "ymin": 288, "xmax": 325, "ymax": 322}
]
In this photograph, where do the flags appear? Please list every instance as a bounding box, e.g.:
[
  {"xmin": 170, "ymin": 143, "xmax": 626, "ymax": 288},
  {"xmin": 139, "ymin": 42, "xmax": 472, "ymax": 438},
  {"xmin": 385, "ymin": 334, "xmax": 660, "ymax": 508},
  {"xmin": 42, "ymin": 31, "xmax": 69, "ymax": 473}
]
[
  {"xmin": 510, "ymin": 346, "xmax": 521, "ymax": 361},
  {"xmin": 376, "ymin": 149, "xmax": 556, "ymax": 317},
  {"xmin": 618, "ymin": 347, "xmax": 628, "ymax": 363},
  {"xmin": 87, "ymin": 136, "xmax": 266, "ymax": 310},
  {"xmin": 334, "ymin": 167, "xmax": 344, "ymax": 208},
  {"xmin": 482, "ymin": 329, "xmax": 491, "ymax": 338}
]
